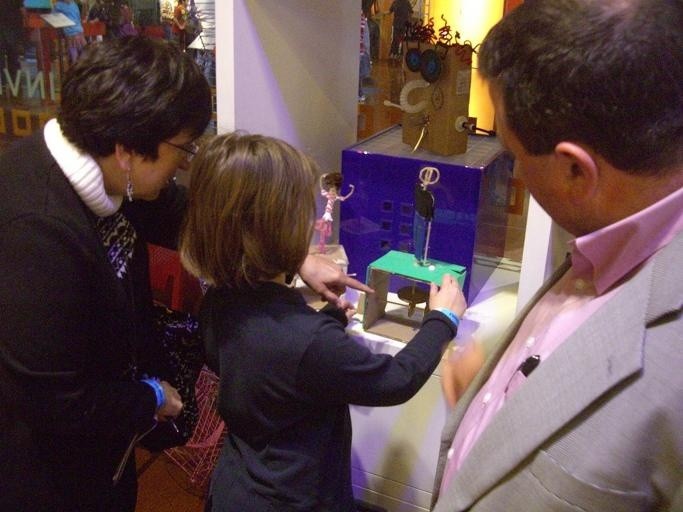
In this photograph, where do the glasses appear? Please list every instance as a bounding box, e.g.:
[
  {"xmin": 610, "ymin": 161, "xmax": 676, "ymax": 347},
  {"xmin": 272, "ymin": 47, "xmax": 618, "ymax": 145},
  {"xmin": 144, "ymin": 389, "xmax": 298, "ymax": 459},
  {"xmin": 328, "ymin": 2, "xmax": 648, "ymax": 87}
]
[{"xmin": 162, "ymin": 139, "xmax": 200, "ymax": 162}]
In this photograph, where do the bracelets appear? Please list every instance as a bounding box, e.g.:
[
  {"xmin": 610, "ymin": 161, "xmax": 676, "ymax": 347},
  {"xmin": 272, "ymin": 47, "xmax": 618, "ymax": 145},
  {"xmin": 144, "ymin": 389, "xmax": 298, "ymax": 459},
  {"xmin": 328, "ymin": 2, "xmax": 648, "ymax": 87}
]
[
  {"xmin": 432, "ymin": 307, "xmax": 459, "ymax": 327},
  {"xmin": 142, "ymin": 379, "xmax": 164, "ymax": 410}
]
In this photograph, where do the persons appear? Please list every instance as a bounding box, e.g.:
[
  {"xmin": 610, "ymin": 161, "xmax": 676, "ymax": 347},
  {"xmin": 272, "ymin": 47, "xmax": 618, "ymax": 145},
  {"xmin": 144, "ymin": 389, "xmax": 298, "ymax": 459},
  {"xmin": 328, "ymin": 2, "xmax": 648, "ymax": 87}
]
[
  {"xmin": 1, "ymin": 34, "xmax": 375, "ymax": 512},
  {"xmin": 429, "ymin": 0, "xmax": 683, "ymax": 512},
  {"xmin": 178, "ymin": 132, "xmax": 467, "ymax": 512},
  {"xmin": 389, "ymin": 0, "xmax": 414, "ymax": 60},
  {"xmin": 50, "ymin": 0, "xmax": 216, "ymax": 86},
  {"xmin": 359, "ymin": 9, "xmax": 373, "ymax": 102}
]
